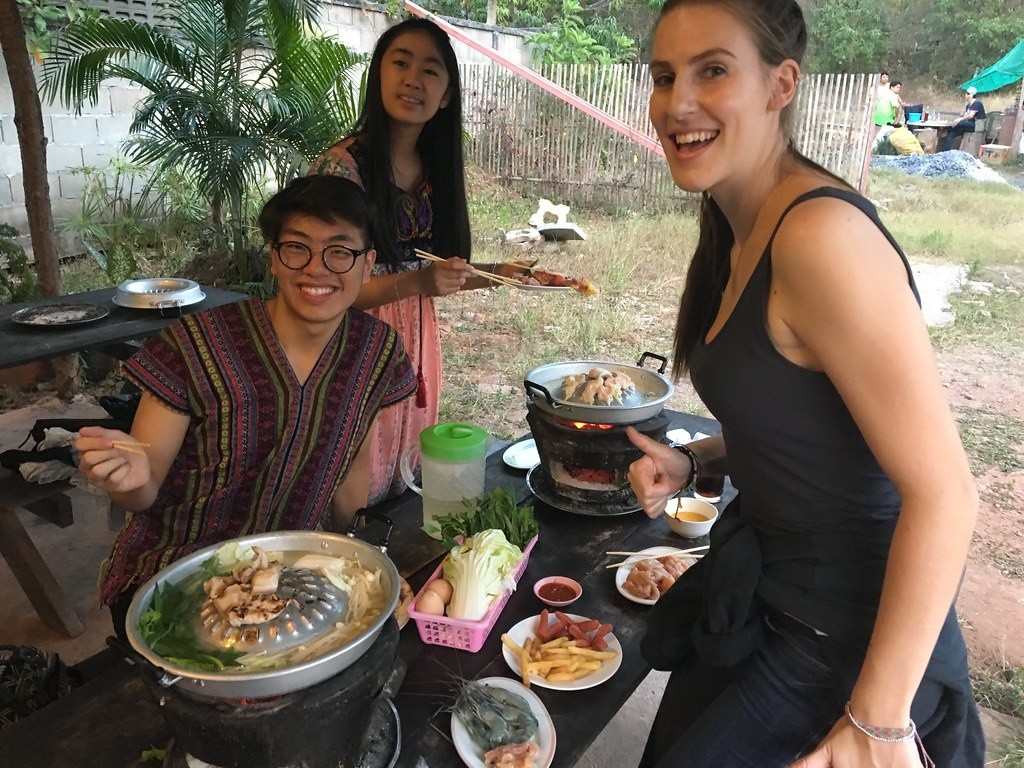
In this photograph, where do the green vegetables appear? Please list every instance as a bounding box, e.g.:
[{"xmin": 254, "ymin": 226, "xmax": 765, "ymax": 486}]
[{"xmin": 432, "ymin": 486, "xmax": 540, "ymax": 553}]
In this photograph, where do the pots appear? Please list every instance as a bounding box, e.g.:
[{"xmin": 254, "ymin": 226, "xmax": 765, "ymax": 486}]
[
  {"xmin": 525, "ymin": 352, "xmax": 673, "ymax": 422},
  {"xmin": 105, "ymin": 508, "xmax": 400, "ymax": 700}
]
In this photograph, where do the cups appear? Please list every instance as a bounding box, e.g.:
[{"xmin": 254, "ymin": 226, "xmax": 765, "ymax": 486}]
[{"xmin": 695, "ymin": 474, "xmax": 725, "ymax": 502}]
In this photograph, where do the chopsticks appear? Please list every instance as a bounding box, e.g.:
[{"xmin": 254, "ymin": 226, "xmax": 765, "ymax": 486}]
[
  {"xmin": 70, "ymin": 432, "xmax": 151, "ymax": 456},
  {"xmin": 605, "ymin": 546, "xmax": 712, "ymax": 568},
  {"xmin": 414, "ymin": 247, "xmax": 522, "ymax": 292}
]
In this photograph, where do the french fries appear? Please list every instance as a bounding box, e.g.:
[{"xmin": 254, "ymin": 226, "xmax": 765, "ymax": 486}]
[{"xmin": 501, "ymin": 633, "xmax": 618, "ymax": 688}]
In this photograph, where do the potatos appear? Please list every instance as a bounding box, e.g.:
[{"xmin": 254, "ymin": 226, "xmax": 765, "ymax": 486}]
[{"xmin": 416, "ymin": 578, "xmax": 453, "ymax": 617}]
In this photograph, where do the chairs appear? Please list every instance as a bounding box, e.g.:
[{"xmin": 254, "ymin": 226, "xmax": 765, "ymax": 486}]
[{"xmin": 904, "ymin": 104, "xmax": 923, "ymax": 124}]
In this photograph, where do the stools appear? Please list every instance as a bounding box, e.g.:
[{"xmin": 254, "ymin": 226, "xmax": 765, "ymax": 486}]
[
  {"xmin": 913, "ymin": 129, "xmax": 937, "ymax": 152},
  {"xmin": 959, "ymin": 132, "xmax": 986, "ymax": 156}
]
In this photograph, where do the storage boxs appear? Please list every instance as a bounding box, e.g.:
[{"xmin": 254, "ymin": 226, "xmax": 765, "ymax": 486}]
[{"xmin": 978, "ymin": 144, "xmax": 1013, "ymax": 164}]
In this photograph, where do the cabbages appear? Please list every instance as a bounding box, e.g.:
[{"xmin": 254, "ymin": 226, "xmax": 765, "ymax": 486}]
[{"xmin": 440, "ymin": 527, "xmax": 526, "ymax": 622}]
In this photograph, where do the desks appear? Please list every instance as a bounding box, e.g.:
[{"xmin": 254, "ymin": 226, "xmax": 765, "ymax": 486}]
[
  {"xmin": 906, "ymin": 120, "xmax": 954, "ymax": 136},
  {"xmin": 0, "ymin": 285, "xmax": 250, "ymax": 369}
]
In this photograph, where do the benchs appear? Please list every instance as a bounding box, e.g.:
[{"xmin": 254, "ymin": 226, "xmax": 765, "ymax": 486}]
[
  {"xmin": 71, "ymin": 481, "xmax": 423, "ymax": 680},
  {"xmin": 0, "ymin": 464, "xmax": 85, "ymax": 637}
]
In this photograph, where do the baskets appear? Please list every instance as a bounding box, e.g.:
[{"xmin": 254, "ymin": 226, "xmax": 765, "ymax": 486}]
[{"xmin": 407, "ymin": 535, "xmax": 538, "ymax": 653}]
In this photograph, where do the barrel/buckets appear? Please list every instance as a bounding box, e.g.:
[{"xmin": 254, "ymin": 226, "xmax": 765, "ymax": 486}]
[
  {"xmin": 904, "ymin": 103, "xmax": 922, "ymax": 121},
  {"xmin": 909, "ymin": 113, "xmax": 921, "ymax": 123}
]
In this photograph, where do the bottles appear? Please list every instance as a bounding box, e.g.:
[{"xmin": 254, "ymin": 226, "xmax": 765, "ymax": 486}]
[{"xmin": 923, "ymin": 106, "xmax": 940, "ymax": 121}]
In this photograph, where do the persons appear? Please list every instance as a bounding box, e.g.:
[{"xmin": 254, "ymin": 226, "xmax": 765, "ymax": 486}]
[
  {"xmin": 942, "ymin": 86, "xmax": 986, "ymax": 151},
  {"xmin": 305, "ymin": 17, "xmax": 534, "ymax": 508},
  {"xmin": 626, "ymin": 0, "xmax": 987, "ymax": 768},
  {"xmin": 75, "ymin": 175, "xmax": 419, "ymax": 640},
  {"xmin": 869, "ymin": 72, "xmax": 906, "ymax": 107},
  {"xmin": 873, "ymin": 80, "xmax": 904, "ymax": 128}
]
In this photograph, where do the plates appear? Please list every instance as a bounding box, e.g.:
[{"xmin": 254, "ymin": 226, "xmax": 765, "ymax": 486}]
[
  {"xmin": 505, "ymin": 278, "xmax": 572, "ymax": 292},
  {"xmin": 450, "ymin": 677, "xmax": 557, "ymax": 768},
  {"xmin": 503, "ymin": 436, "xmax": 542, "ymax": 470},
  {"xmin": 616, "ymin": 546, "xmax": 699, "ymax": 604},
  {"xmin": 12, "ymin": 304, "xmax": 108, "ymax": 324},
  {"xmin": 502, "ymin": 613, "xmax": 623, "ymax": 690}
]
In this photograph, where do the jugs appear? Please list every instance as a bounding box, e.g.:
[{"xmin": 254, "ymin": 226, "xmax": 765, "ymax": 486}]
[{"xmin": 400, "ymin": 423, "xmax": 496, "ymax": 541}]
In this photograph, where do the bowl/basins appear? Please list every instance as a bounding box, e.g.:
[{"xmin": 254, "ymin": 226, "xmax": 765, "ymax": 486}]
[
  {"xmin": 534, "ymin": 575, "xmax": 582, "ymax": 606},
  {"xmin": 664, "ymin": 497, "xmax": 718, "ymax": 539}
]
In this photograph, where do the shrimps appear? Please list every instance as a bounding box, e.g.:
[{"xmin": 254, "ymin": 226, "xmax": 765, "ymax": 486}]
[{"xmin": 427, "ymin": 655, "xmax": 539, "ymax": 750}]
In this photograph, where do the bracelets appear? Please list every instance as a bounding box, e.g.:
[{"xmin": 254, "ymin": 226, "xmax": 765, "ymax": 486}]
[
  {"xmin": 845, "ymin": 702, "xmax": 936, "ymax": 768},
  {"xmin": 394, "ymin": 272, "xmax": 403, "ymax": 301},
  {"xmin": 668, "ymin": 442, "xmax": 701, "ymax": 499},
  {"xmin": 488, "ymin": 262, "xmax": 496, "ymax": 292}
]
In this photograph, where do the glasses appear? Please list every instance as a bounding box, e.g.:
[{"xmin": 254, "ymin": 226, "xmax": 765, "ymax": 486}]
[{"xmin": 273, "ymin": 240, "xmax": 368, "ymax": 274}]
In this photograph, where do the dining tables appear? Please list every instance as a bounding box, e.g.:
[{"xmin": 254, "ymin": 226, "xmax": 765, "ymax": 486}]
[{"xmin": 0, "ymin": 409, "xmax": 740, "ymax": 768}]
